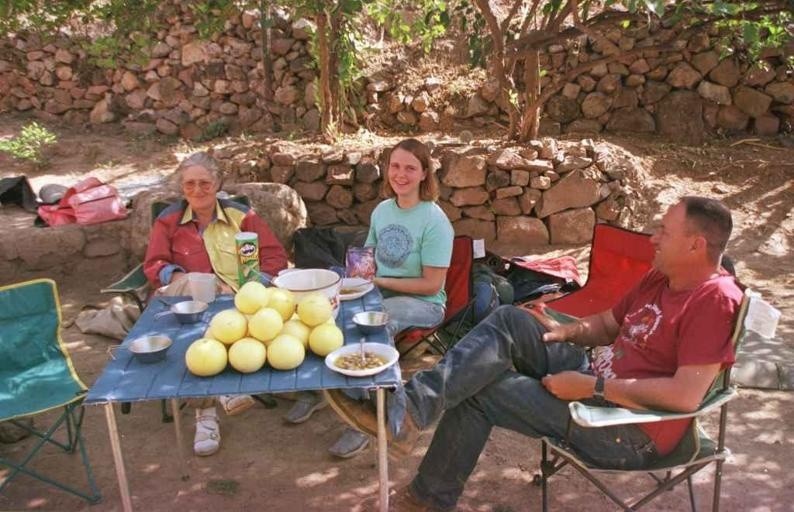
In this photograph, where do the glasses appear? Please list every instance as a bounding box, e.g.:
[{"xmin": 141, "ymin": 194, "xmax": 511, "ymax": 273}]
[{"xmin": 180, "ymin": 178, "xmax": 219, "ymax": 191}]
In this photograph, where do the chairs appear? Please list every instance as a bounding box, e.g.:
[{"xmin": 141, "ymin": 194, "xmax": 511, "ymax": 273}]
[
  {"xmin": 0, "ymin": 278, "xmax": 101, "ymax": 505},
  {"xmin": 542, "ymin": 276, "xmax": 751, "ymax": 512},
  {"xmin": 102, "ymin": 196, "xmax": 277, "ymax": 420},
  {"xmin": 537, "ymin": 224, "xmax": 655, "ymax": 318},
  {"xmin": 395, "ymin": 235, "xmax": 473, "ymax": 358}
]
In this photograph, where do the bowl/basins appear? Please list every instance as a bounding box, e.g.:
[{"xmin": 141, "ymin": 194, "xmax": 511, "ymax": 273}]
[
  {"xmin": 353, "ymin": 310, "xmax": 391, "ymax": 334},
  {"xmin": 326, "ymin": 343, "xmax": 401, "ymax": 377},
  {"xmin": 169, "ymin": 300, "xmax": 209, "ymax": 326},
  {"xmin": 268, "ymin": 269, "xmax": 339, "ymax": 324},
  {"xmin": 131, "ymin": 335, "xmax": 173, "ymax": 361}
]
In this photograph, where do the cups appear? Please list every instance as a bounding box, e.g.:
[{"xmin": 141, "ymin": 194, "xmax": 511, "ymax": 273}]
[{"xmin": 189, "ymin": 271, "xmax": 218, "ymax": 302}]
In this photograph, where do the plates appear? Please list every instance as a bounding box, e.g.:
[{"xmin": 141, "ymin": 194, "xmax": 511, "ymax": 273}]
[{"xmin": 339, "ymin": 277, "xmax": 375, "ymax": 300}]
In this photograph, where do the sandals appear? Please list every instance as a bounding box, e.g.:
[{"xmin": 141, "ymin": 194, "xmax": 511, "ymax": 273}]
[
  {"xmin": 220, "ymin": 391, "xmax": 254, "ymax": 417},
  {"xmin": 193, "ymin": 412, "xmax": 219, "ymax": 458}
]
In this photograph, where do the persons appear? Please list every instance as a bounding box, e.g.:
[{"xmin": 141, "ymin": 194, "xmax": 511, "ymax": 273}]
[
  {"xmin": 323, "ymin": 196, "xmax": 744, "ymax": 512},
  {"xmin": 283, "ymin": 139, "xmax": 454, "ymax": 458},
  {"xmin": 143, "ymin": 153, "xmax": 289, "ymax": 456}
]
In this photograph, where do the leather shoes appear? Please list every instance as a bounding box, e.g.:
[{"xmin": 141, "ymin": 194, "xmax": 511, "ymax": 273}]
[{"xmin": 325, "ymin": 386, "xmax": 418, "ymax": 463}]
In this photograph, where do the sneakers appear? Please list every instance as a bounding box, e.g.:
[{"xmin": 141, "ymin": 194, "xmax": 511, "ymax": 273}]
[
  {"xmin": 283, "ymin": 392, "xmax": 326, "ymax": 426},
  {"xmin": 329, "ymin": 428, "xmax": 371, "ymax": 461},
  {"xmin": 371, "ymin": 485, "xmax": 442, "ymax": 511}
]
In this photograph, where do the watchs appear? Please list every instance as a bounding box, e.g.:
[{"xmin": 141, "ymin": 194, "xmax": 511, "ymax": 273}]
[{"xmin": 593, "ymin": 377, "xmax": 605, "ymax": 404}]
